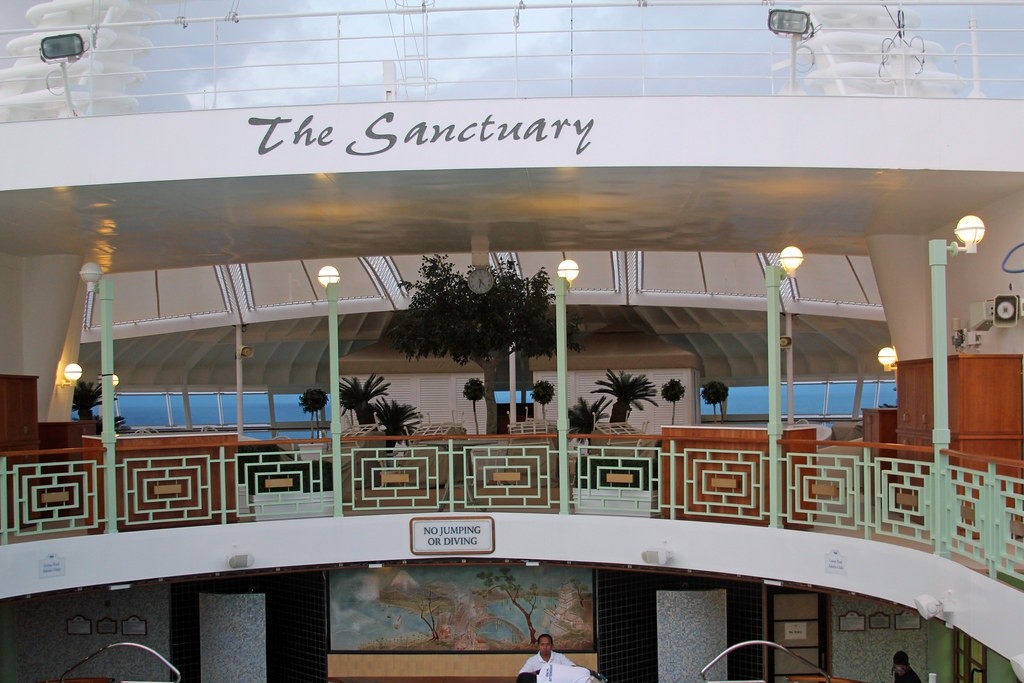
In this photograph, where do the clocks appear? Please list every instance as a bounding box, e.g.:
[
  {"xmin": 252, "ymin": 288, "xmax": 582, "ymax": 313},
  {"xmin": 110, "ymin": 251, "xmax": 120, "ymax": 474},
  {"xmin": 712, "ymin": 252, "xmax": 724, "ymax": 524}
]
[{"xmin": 467, "ymin": 269, "xmax": 494, "ymax": 295}]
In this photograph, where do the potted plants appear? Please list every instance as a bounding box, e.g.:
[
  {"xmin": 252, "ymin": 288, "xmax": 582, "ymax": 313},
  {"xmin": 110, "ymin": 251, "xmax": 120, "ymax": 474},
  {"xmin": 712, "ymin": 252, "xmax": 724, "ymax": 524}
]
[{"xmin": 298, "ymin": 388, "xmax": 330, "ymax": 461}]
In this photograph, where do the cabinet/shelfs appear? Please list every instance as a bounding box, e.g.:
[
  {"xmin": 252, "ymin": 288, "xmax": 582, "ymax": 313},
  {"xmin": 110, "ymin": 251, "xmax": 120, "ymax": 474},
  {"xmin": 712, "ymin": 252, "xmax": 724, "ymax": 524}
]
[
  {"xmin": 861, "ymin": 408, "xmax": 898, "ymax": 505},
  {"xmin": 660, "ymin": 425, "xmax": 817, "ymax": 529},
  {"xmin": 81, "ymin": 432, "xmax": 239, "ymax": 536},
  {"xmin": 39, "ymin": 421, "xmax": 98, "ymax": 519},
  {"xmin": 1, "ymin": 375, "xmax": 42, "ymax": 532},
  {"xmin": 895, "ymin": 354, "xmax": 1023, "ymax": 540}
]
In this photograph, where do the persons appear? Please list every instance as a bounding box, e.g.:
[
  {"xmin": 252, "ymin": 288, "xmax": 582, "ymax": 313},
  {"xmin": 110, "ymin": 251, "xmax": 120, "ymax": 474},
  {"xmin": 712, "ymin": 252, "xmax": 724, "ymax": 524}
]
[
  {"xmin": 893, "ymin": 650, "xmax": 921, "ymax": 683},
  {"xmin": 515, "ymin": 634, "xmax": 594, "ymax": 683}
]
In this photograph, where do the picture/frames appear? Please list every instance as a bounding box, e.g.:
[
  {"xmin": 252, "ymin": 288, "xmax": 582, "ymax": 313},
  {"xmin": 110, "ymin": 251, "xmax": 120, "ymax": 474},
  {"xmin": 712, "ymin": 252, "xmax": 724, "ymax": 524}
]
[{"xmin": 325, "ymin": 566, "xmax": 599, "ymax": 655}]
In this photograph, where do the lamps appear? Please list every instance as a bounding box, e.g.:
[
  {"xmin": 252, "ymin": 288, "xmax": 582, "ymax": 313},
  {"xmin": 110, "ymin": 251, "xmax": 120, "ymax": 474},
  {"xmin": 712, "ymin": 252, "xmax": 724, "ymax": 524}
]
[
  {"xmin": 768, "ymin": 9, "xmax": 823, "ymax": 95},
  {"xmin": 878, "ymin": 347, "xmax": 898, "ymax": 372},
  {"xmin": 56, "ymin": 363, "xmax": 84, "ymax": 388},
  {"xmin": 39, "ymin": 33, "xmax": 91, "ymax": 118}
]
